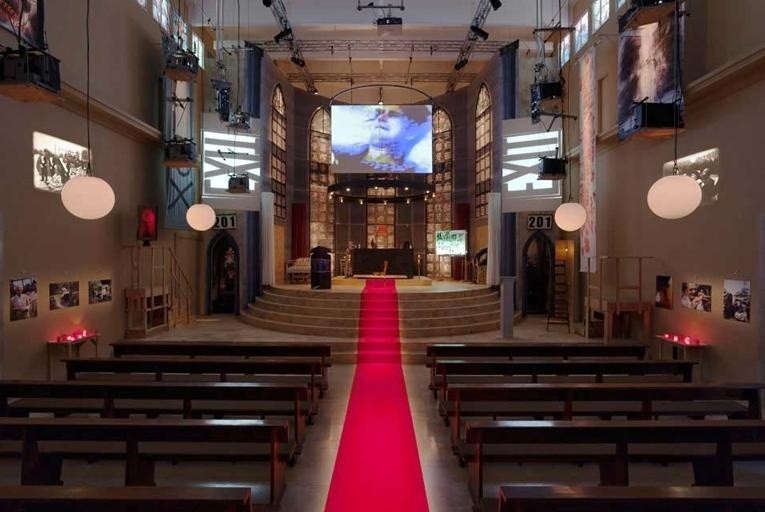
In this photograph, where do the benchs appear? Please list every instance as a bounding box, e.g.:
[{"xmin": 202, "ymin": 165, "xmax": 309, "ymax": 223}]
[{"xmin": 286, "ymin": 256, "xmax": 312, "ymax": 285}]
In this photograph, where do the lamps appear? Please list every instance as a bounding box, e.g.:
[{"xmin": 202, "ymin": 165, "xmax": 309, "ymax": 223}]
[
  {"xmin": 60, "ymin": 0, "xmax": 116, "ymax": 220},
  {"xmin": 262, "ymin": 0, "xmax": 318, "ymax": 94},
  {"xmin": 646, "ymin": 1, "xmax": 703, "ymax": 221},
  {"xmin": 454, "ymin": 0, "xmax": 502, "ymax": 70},
  {"xmin": 185, "ymin": 0, "xmax": 217, "ymax": 232},
  {"xmin": 321, "ymin": 86, "xmax": 438, "ymax": 207},
  {"xmin": 554, "ymin": 1, "xmax": 586, "ymax": 232}
]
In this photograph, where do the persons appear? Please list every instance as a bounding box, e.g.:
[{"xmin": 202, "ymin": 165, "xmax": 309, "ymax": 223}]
[
  {"xmin": 30, "ymin": 133, "xmax": 92, "ymax": 192},
  {"xmin": 332, "ymin": 106, "xmax": 432, "ymax": 172},
  {"xmin": 9, "ymin": 278, "xmax": 112, "ymax": 319},
  {"xmin": 653, "ymin": 275, "xmax": 751, "ymax": 322}
]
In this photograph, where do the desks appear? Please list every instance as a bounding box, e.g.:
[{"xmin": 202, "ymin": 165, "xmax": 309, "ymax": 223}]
[
  {"xmin": 44, "ymin": 333, "xmax": 100, "ymax": 380},
  {"xmin": 585, "ymin": 296, "xmax": 652, "ymax": 343},
  {"xmin": 656, "ymin": 334, "xmax": 711, "ymax": 381}
]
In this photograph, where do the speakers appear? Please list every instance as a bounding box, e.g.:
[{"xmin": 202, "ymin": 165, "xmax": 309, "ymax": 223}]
[{"xmin": 135, "ymin": 205, "xmax": 158, "ymax": 246}]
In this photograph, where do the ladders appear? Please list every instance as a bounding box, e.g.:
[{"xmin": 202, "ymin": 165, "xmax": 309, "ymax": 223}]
[{"xmin": 546, "ymin": 259, "xmax": 572, "ymax": 336}]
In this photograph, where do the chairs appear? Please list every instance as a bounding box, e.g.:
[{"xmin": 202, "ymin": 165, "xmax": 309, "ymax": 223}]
[
  {"xmin": 498, "ymin": 486, "xmax": 764, "ymax": 510},
  {"xmin": 425, "ymin": 342, "xmax": 763, "ymax": 468},
  {"xmin": 2, "ymin": 343, "xmax": 334, "ymax": 465},
  {"xmin": 462, "ymin": 420, "xmax": 764, "ymax": 505},
  {"xmin": 2, "ymin": 417, "xmax": 297, "ymax": 508},
  {"xmin": 2, "ymin": 486, "xmax": 252, "ymax": 511}
]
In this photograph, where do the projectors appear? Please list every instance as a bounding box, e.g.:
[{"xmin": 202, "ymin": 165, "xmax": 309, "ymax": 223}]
[{"xmin": 377, "ymin": 18, "xmax": 402, "ymax": 36}]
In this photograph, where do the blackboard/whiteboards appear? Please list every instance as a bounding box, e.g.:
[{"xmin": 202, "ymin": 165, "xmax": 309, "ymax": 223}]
[{"xmin": 434, "ymin": 229, "xmax": 468, "ymax": 256}]
